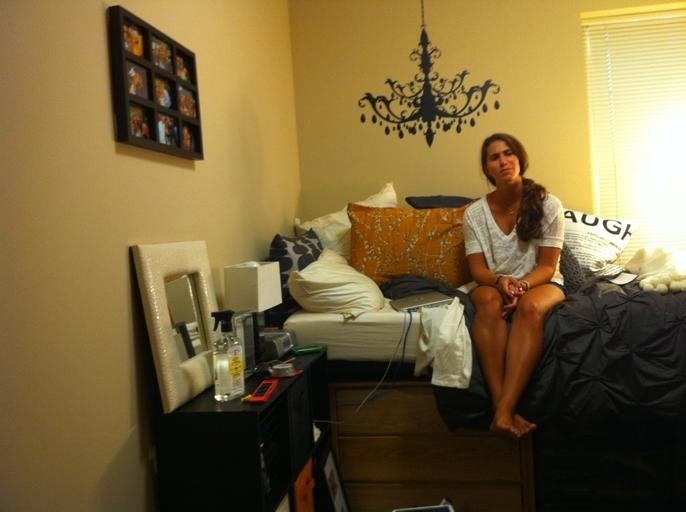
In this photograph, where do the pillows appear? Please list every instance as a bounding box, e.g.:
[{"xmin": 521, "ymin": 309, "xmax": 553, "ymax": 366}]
[
  {"xmin": 288, "ymin": 247, "xmax": 389, "ymax": 316},
  {"xmin": 290, "ymin": 184, "xmax": 400, "ymax": 267},
  {"xmin": 562, "ymin": 205, "xmax": 635, "ymax": 279},
  {"xmin": 405, "ymin": 195, "xmax": 472, "ymax": 208},
  {"xmin": 269, "ymin": 229, "xmax": 323, "ymax": 303},
  {"xmin": 343, "ymin": 198, "xmax": 475, "ymax": 290}
]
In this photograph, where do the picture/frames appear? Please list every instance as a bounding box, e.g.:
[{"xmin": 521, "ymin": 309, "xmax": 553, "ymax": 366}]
[{"xmin": 104, "ymin": 6, "xmax": 205, "ymax": 164}]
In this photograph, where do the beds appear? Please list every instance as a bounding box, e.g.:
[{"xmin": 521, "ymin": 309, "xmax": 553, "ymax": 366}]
[{"xmin": 268, "ymin": 274, "xmax": 686, "ymax": 365}]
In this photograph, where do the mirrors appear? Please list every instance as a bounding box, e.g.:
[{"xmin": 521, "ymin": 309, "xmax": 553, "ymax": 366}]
[{"xmin": 128, "ymin": 237, "xmax": 231, "ymax": 418}]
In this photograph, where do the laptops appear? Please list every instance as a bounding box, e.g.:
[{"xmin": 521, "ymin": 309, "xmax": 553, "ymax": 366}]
[{"xmin": 390, "ymin": 291, "xmax": 453, "ymax": 313}]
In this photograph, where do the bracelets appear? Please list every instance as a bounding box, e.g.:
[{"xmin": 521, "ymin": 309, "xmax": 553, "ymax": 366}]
[
  {"xmin": 519, "ymin": 280, "xmax": 529, "ymax": 291},
  {"xmin": 495, "ymin": 276, "xmax": 503, "ymax": 289}
]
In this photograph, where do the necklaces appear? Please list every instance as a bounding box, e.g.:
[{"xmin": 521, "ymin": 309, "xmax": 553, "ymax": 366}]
[{"xmin": 500, "ymin": 201, "xmax": 520, "ymax": 215}]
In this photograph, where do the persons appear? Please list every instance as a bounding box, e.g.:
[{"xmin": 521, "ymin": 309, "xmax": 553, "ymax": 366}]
[{"xmin": 463, "ymin": 134, "xmax": 565, "ymax": 442}]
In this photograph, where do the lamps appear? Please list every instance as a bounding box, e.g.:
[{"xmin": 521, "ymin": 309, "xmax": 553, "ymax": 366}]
[
  {"xmin": 359, "ymin": 1, "xmax": 502, "ymax": 147},
  {"xmin": 222, "ymin": 259, "xmax": 284, "ymax": 376}
]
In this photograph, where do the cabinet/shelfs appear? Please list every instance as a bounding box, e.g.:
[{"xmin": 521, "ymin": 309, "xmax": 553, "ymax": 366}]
[
  {"xmin": 332, "ymin": 359, "xmax": 536, "ymax": 511},
  {"xmin": 149, "ymin": 341, "xmax": 332, "ymax": 511}
]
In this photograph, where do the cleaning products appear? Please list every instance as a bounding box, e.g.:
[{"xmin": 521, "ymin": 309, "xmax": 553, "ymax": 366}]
[{"xmin": 210, "ymin": 309, "xmax": 247, "ymax": 403}]
